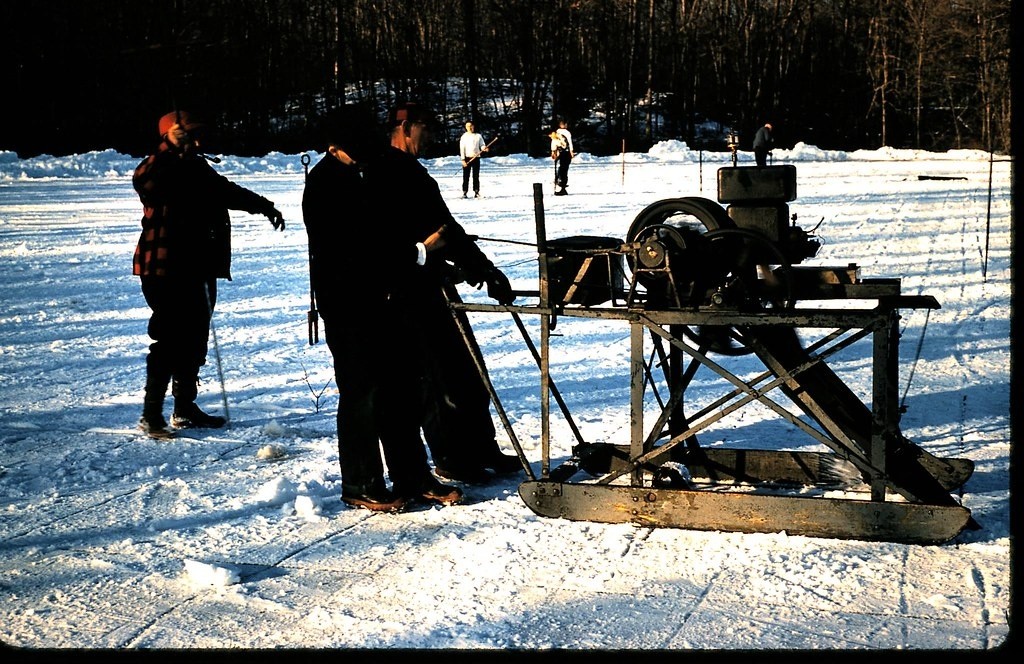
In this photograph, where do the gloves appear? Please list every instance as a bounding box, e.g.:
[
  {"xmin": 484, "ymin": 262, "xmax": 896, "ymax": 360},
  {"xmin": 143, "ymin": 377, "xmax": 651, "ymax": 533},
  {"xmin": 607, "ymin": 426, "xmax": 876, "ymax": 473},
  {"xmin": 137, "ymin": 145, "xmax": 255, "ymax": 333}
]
[{"xmin": 486, "ymin": 268, "xmax": 516, "ymax": 303}]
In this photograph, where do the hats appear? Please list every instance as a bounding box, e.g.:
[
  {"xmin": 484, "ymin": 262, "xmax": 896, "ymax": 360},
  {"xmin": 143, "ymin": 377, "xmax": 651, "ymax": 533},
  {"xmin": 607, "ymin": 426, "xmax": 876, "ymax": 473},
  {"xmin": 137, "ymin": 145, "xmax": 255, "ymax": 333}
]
[
  {"xmin": 159, "ymin": 108, "xmax": 205, "ymax": 137},
  {"xmin": 388, "ymin": 102, "xmax": 446, "ymax": 132}
]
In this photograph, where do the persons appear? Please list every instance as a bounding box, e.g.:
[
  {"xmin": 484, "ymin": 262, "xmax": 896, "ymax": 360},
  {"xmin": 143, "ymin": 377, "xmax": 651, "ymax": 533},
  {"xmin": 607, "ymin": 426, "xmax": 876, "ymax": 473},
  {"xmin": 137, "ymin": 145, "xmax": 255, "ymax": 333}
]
[
  {"xmin": 302, "ymin": 109, "xmax": 462, "ymax": 512},
  {"xmin": 362, "ymin": 101, "xmax": 523, "ymax": 486},
  {"xmin": 459, "ymin": 122, "xmax": 489, "ymax": 198},
  {"xmin": 753, "ymin": 122, "xmax": 774, "ymax": 166},
  {"xmin": 548, "ymin": 121, "xmax": 573, "ymax": 195},
  {"xmin": 131, "ymin": 110, "xmax": 285, "ymax": 440}
]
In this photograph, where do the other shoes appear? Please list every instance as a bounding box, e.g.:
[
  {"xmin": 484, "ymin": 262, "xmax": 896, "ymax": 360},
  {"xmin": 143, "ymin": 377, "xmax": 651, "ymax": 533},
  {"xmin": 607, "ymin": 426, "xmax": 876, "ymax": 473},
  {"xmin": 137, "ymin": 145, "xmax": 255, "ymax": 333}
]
[
  {"xmin": 480, "ymin": 441, "xmax": 526, "ymax": 475},
  {"xmin": 139, "ymin": 414, "xmax": 177, "ymax": 438},
  {"xmin": 391, "ymin": 480, "xmax": 464, "ymax": 504},
  {"xmin": 341, "ymin": 485, "xmax": 404, "ymax": 512},
  {"xmin": 170, "ymin": 404, "xmax": 226, "ymax": 430},
  {"xmin": 436, "ymin": 464, "xmax": 493, "ymax": 485}
]
